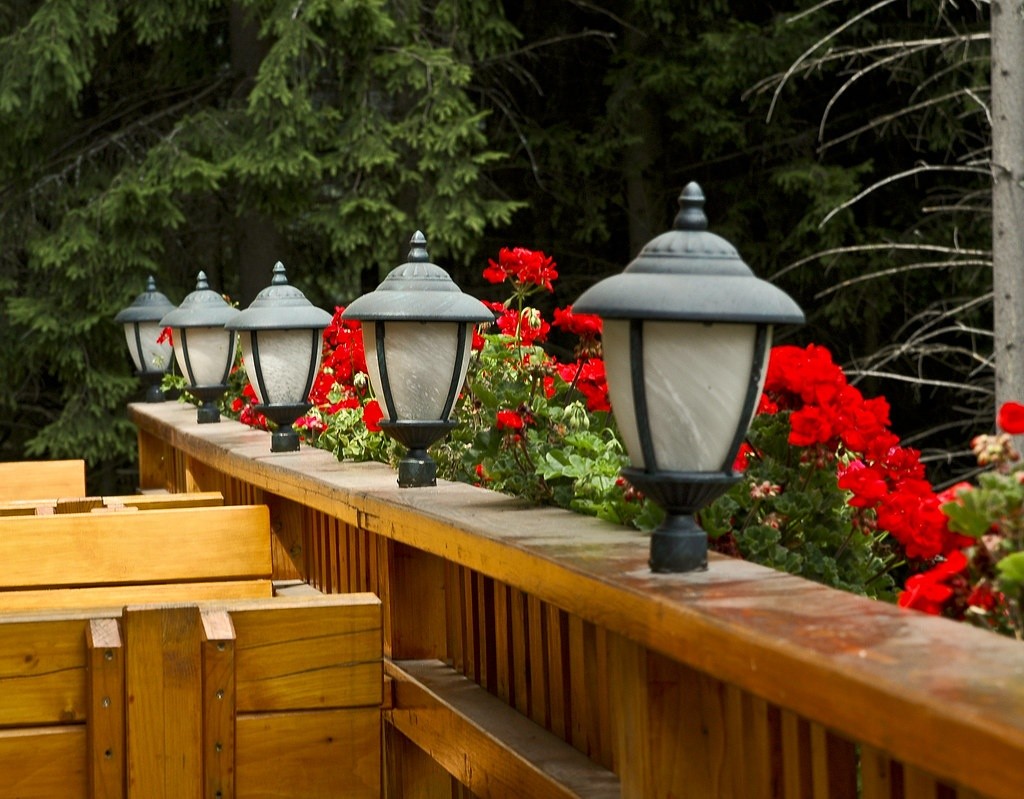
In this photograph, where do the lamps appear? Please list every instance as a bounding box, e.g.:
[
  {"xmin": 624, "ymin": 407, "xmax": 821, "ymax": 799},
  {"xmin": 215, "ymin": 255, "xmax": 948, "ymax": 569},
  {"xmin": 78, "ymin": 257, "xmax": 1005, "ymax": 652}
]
[
  {"xmin": 115, "ymin": 276, "xmax": 172, "ymax": 402},
  {"xmin": 341, "ymin": 227, "xmax": 498, "ymax": 490},
  {"xmin": 571, "ymin": 182, "xmax": 805, "ymax": 571},
  {"xmin": 222, "ymin": 260, "xmax": 332, "ymax": 452},
  {"xmin": 159, "ymin": 271, "xmax": 242, "ymax": 422}
]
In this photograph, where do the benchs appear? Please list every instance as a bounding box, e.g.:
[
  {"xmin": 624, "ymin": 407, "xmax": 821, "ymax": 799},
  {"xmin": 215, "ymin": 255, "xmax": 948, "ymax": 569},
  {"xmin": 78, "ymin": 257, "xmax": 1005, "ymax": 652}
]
[{"xmin": 0, "ymin": 460, "xmax": 394, "ymax": 799}]
